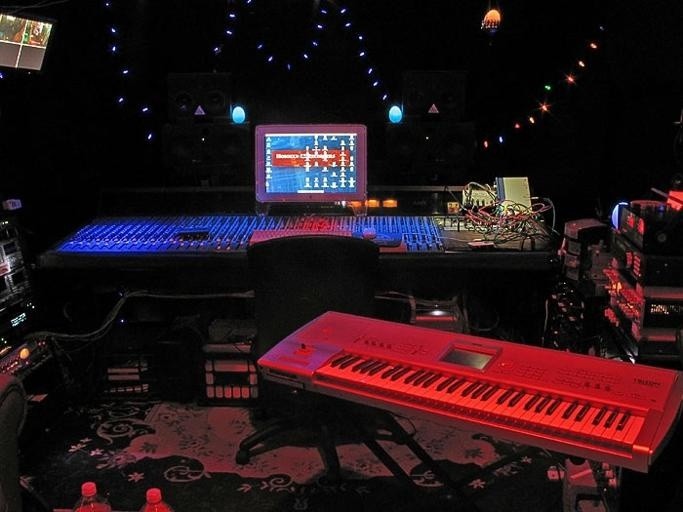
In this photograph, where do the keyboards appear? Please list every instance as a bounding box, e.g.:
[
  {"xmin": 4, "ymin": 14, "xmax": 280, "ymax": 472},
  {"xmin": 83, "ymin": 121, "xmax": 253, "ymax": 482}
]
[{"xmin": 250, "ymin": 229, "xmax": 351, "ymax": 248}]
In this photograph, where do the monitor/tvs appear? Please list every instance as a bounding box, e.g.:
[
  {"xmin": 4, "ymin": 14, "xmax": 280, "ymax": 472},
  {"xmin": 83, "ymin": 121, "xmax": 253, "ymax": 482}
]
[
  {"xmin": 0, "ymin": 7, "xmax": 59, "ymax": 80},
  {"xmin": 254, "ymin": 124, "xmax": 368, "ymax": 218}
]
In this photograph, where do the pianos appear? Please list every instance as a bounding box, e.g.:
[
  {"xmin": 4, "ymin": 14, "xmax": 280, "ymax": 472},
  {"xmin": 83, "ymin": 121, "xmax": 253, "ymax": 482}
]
[{"xmin": 256, "ymin": 310, "xmax": 683, "ymax": 474}]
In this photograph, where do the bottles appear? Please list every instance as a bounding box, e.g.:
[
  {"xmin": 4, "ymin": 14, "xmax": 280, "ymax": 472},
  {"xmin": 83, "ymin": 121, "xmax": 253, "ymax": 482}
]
[
  {"xmin": 72, "ymin": 482, "xmax": 112, "ymax": 512},
  {"xmin": 139, "ymin": 488, "xmax": 173, "ymax": 512}
]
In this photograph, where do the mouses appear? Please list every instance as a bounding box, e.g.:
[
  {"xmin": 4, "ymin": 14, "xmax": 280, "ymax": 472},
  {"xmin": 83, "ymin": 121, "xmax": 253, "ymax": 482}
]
[{"xmin": 364, "ymin": 227, "xmax": 377, "ymax": 239}]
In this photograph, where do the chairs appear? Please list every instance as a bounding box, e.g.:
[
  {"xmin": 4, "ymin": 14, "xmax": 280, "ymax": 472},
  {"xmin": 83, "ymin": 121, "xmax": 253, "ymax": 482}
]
[{"xmin": 235, "ymin": 234, "xmax": 408, "ymax": 490}]
